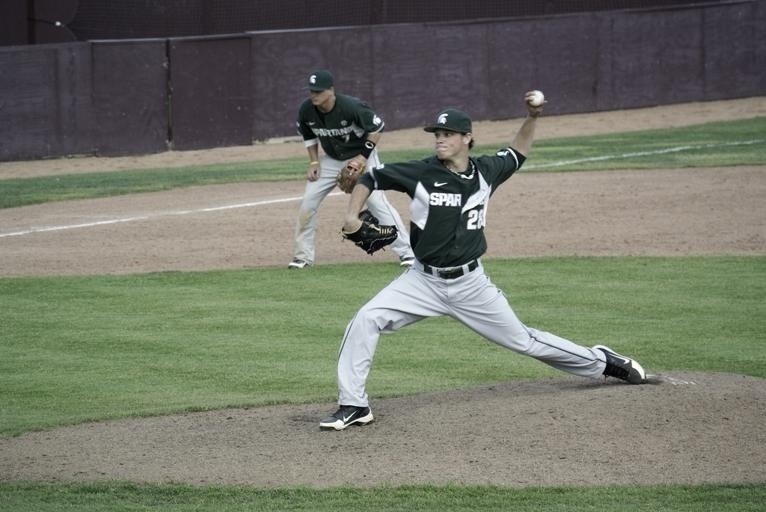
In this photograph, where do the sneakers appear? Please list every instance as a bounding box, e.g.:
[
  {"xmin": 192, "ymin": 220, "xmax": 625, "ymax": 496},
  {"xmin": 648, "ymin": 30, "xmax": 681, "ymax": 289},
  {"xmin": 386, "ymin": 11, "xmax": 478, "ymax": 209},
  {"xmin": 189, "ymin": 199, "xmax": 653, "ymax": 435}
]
[
  {"xmin": 287, "ymin": 258, "xmax": 310, "ymax": 270},
  {"xmin": 318, "ymin": 403, "xmax": 376, "ymax": 432},
  {"xmin": 592, "ymin": 344, "xmax": 647, "ymax": 385},
  {"xmin": 399, "ymin": 255, "xmax": 416, "ymax": 268}
]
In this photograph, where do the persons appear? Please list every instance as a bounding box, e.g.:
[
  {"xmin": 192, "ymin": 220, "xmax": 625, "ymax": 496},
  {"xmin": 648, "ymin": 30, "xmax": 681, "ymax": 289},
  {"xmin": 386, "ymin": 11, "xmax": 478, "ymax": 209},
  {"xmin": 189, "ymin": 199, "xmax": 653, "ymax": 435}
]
[
  {"xmin": 319, "ymin": 90, "xmax": 646, "ymax": 431},
  {"xmin": 288, "ymin": 69, "xmax": 416, "ymax": 270}
]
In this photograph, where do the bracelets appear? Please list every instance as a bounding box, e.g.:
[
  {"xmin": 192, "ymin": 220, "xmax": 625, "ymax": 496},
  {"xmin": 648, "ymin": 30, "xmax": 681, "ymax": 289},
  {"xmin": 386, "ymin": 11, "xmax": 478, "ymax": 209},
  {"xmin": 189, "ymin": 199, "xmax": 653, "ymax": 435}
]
[{"xmin": 310, "ymin": 161, "xmax": 320, "ymax": 165}]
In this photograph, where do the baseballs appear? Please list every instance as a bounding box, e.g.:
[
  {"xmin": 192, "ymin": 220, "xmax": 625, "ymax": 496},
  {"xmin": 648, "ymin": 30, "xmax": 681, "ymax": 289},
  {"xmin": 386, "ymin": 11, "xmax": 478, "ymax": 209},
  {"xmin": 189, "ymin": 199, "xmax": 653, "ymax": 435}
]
[{"xmin": 529, "ymin": 90, "xmax": 544, "ymax": 107}]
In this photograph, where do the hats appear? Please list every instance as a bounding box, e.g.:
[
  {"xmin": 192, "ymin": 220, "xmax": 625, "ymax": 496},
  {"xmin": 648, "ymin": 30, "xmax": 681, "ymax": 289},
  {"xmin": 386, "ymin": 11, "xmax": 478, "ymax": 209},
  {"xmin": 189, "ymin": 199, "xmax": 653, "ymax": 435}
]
[
  {"xmin": 302, "ymin": 69, "xmax": 335, "ymax": 93},
  {"xmin": 423, "ymin": 107, "xmax": 473, "ymax": 134}
]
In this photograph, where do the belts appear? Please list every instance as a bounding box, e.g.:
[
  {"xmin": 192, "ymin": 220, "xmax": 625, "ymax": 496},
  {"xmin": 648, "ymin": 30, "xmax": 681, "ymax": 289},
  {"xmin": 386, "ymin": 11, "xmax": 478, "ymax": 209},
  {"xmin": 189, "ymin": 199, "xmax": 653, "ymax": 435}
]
[{"xmin": 423, "ymin": 260, "xmax": 479, "ymax": 281}]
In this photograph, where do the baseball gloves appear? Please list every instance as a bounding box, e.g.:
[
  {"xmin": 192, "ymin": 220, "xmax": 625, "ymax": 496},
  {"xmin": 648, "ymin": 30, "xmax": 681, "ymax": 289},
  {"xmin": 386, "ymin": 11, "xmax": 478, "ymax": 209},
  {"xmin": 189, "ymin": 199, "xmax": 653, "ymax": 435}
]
[
  {"xmin": 339, "ymin": 210, "xmax": 397, "ymax": 255},
  {"xmin": 336, "ymin": 154, "xmax": 366, "ymax": 194}
]
